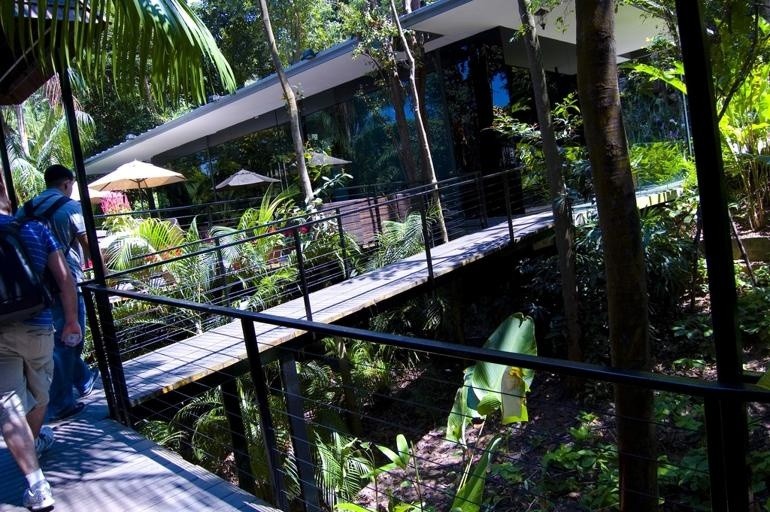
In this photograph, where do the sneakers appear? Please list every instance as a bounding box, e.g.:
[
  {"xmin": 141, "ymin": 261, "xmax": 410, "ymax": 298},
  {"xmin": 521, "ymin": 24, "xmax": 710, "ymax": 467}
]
[
  {"xmin": 79, "ymin": 367, "xmax": 99, "ymax": 397},
  {"xmin": 47, "ymin": 403, "xmax": 85, "ymax": 422},
  {"xmin": 33, "ymin": 427, "xmax": 54, "ymax": 459},
  {"xmin": 22, "ymin": 480, "xmax": 55, "ymax": 511}
]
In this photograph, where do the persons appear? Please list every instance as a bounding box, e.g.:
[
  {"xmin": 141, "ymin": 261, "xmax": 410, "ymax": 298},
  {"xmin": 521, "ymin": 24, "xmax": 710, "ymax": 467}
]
[
  {"xmin": 1, "ymin": 193, "xmax": 83, "ymax": 510},
  {"xmin": 14, "ymin": 164, "xmax": 101, "ymax": 421}
]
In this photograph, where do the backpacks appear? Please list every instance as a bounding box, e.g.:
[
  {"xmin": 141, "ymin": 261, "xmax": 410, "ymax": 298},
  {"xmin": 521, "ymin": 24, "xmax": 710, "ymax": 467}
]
[
  {"xmin": 23, "ymin": 196, "xmax": 73, "ymax": 256},
  {"xmin": 0, "ymin": 217, "xmax": 54, "ymax": 324}
]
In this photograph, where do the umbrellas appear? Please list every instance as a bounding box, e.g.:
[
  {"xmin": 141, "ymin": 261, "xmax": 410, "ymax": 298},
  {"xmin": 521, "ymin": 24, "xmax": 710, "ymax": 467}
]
[
  {"xmin": 209, "ymin": 168, "xmax": 281, "ymax": 190},
  {"xmin": 289, "ymin": 152, "xmax": 352, "ymax": 167},
  {"xmin": 87, "ymin": 157, "xmax": 188, "ymax": 210}
]
